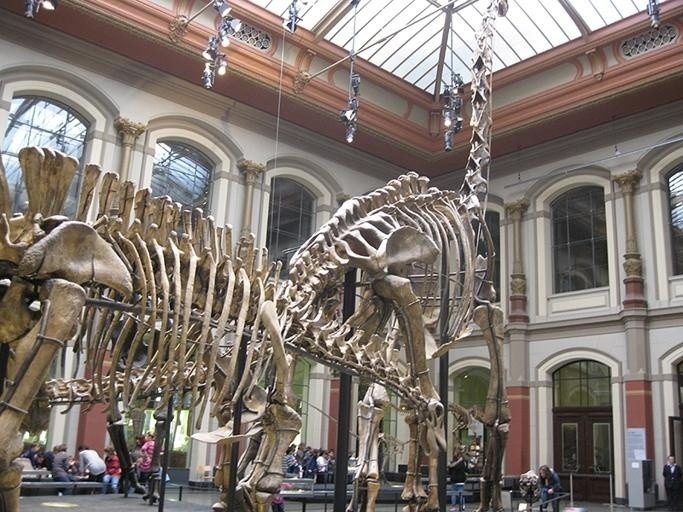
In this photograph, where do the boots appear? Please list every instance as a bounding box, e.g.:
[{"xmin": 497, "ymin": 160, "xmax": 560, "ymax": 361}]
[{"xmin": 449, "ymin": 505, "xmax": 465, "ymax": 512}]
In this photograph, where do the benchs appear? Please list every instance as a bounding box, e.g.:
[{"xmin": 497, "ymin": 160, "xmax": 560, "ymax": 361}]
[{"xmin": 13, "ymin": 458, "xmax": 89, "ymax": 496}]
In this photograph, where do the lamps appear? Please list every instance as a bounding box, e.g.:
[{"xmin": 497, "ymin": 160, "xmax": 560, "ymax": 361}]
[
  {"xmin": 282, "ymin": 0, "xmax": 308, "ymax": 35},
  {"xmin": 339, "ymin": 0, "xmax": 361, "ymax": 143},
  {"xmin": 168, "ymin": 0, "xmax": 243, "ymax": 89},
  {"xmin": 611, "ymin": 116, "xmax": 621, "ymax": 156},
  {"xmin": 441, "ymin": 0, "xmax": 464, "ymax": 153},
  {"xmin": 646, "ymin": 0, "xmax": 661, "ymax": 28},
  {"xmin": 24, "ymin": 0, "xmax": 59, "ymax": 19}
]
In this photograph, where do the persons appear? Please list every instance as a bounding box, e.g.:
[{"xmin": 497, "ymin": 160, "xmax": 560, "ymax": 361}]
[
  {"xmin": 536, "ymin": 464, "xmax": 562, "ymax": 511},
  {"xmin": 19, "ymin": 432, "xmax": 165, "ymax": 495},
  {"xmin": 662, "ymin": 455, "xmax": 681, "ymax": 512},
  {"xmin": 467, "ymin": 441, "xmax": 481, "ymax": 466},
  {"xmin": 446, "ymin": 450, "xmax": 467, "ymax": 512},
  {"xmin": 282, "ymin": 441, "xmax": 358, "ymax": 485}
]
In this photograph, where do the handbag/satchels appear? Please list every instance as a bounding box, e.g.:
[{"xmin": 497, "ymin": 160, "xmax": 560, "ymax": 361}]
[{"xmin": 288, "ymin": 466, "xmax": 299, "ymax": 474}]
